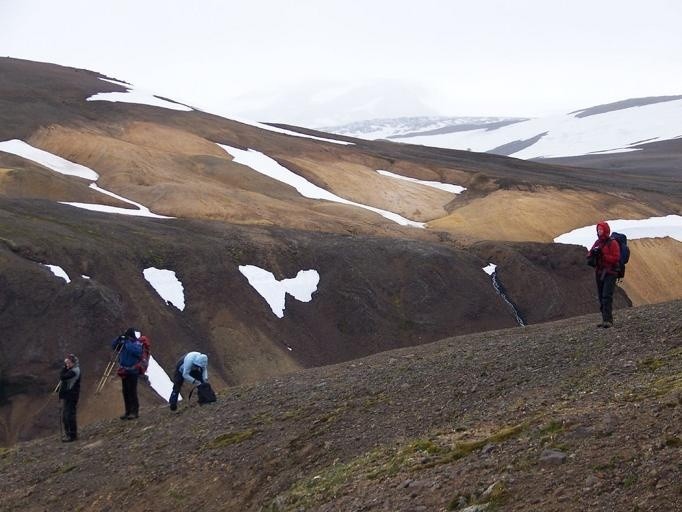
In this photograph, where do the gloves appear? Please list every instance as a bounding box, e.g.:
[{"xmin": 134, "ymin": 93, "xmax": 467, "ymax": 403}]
[{"xmin": 193, "ymin": 379, "xmax": 201, "ymax": 386}]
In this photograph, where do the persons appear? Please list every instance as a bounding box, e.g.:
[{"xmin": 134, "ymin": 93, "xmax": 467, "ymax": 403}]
[
  {"xmin": 168, "ymin": 350, "xmax": 209, "ymax": 411},
  {"xmin": 585, "ymin": 221, "xmax": 621, "ymax": 328},
  {"xmin": 56, "ymin": 353, "xmax": 81, "ymax": 443},
  {"xmin": 111, "ymin": 326, "xmax": 151, "ymax": 420}
]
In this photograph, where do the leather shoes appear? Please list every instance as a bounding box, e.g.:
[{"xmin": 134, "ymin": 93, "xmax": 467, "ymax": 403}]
[
  {"xmin": 127, "ymin": 413, "xmax": 136, "ymax": 419},
  {"xmin": 120, "ymin": 414, "xmax": 127, "ymax": 420},
  {"xmin": 62, "ymin": 435, "xmax": 79, "ymax": 442}
]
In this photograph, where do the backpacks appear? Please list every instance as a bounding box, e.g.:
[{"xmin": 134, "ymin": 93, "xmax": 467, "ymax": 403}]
[
  {"xmin": 188, "ymin": 381, "xmax": 217, "ymax": 406},
  {"xmin": 606, "ymin": 232, "xmax": 630, "ymax": 283},
  {"xmin": 132, "ymin": 335, "xmax": 151, "ymax": 375}
]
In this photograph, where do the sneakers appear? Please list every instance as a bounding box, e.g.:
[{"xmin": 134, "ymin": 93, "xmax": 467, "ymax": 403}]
[
  {"xmin": 596, "ymin": 320, "xmax": 613, "ymax": 329},
  {"xmin": 170, "ymin": 403, "xmax": 178, "ymax": 411}
]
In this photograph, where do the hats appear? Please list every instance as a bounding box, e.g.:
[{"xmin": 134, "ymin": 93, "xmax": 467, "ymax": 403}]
[{"xmin": 124, "ymin": 327, "xmax": 136, "ymax": 339}]
